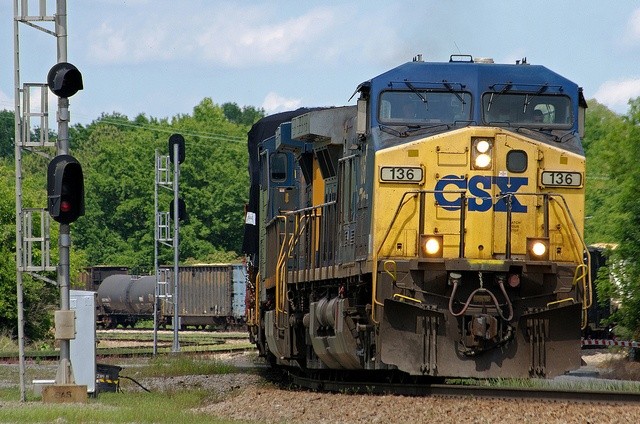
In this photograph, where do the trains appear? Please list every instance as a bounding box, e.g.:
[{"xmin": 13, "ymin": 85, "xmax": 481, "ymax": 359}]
[
  {"xmin": 240, "ymin": 52, "xmax": 629, "ymax": 385},
  {"xmin": 74, "ymin": 262, "xmax": 247, "ymax": 332}
]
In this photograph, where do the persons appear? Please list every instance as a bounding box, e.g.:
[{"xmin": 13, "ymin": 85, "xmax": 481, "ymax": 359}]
[
  {"xmin": 531, "ymin": 108, "xmax": 545, "ymax": 124},
  {"xmin": 400, "ymin": 101, "xmax": 416, "ymax": 119}
]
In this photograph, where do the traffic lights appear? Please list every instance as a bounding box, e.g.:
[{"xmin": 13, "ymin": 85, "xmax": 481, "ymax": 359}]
[
  {"xmin": 47, "ymin": 61, "xmax": 83, "ymax": 97},
  {"xmin": 46, "ymin": 154, "xmax": 85, "ymax": 223}
]
